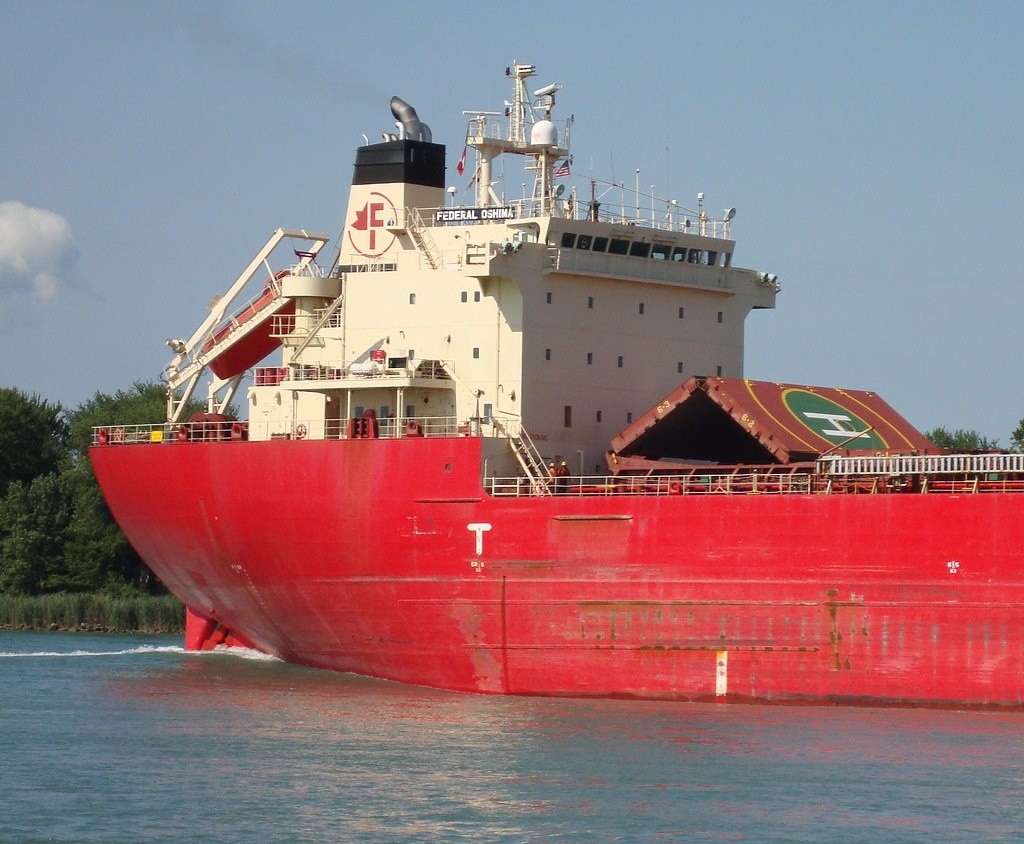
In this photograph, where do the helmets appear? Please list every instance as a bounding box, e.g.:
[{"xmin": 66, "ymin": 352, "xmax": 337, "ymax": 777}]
[
  {"xmin": 548, "ymin": 463, "xmax": 554, "ymax": 467},
  {"xmin": 562, "ymin": 462, "xmax": 567, "ymax": 466}
]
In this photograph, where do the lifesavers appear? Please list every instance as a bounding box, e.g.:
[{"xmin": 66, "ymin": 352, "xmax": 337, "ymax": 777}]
[
  {"xmin": 295, "ymin": 424, "xmax": 307, "ymax": 439},
  {"xmin": 113, "ymin": 429, "xmax": 122, "ymax": 442}
]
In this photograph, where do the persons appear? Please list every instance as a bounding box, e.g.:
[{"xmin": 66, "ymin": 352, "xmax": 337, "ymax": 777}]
[{"xmin": 546, "ymin": 461, "xmax": 571, "ymax": 493}]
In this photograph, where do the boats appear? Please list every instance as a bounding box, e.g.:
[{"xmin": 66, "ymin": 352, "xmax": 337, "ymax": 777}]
[{"xmin": 88, "ymin": 66, "xmax": 1024, "ymax": 714}]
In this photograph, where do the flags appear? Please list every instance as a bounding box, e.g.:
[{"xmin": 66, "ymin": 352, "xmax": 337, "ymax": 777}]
[
  {"xmin": 455, "ymin": 146, "xmax": 467, "ymax": 175},
  {"xmin": 555, "ymin": 160, "xmax": 570, "ymax": 177}
]
